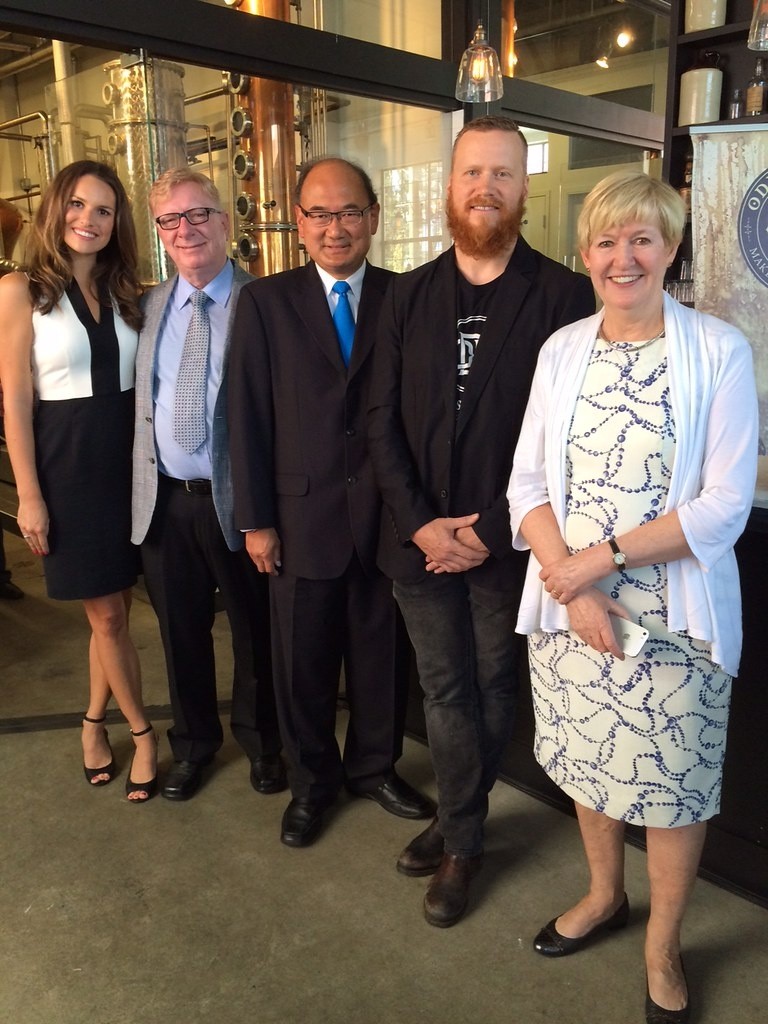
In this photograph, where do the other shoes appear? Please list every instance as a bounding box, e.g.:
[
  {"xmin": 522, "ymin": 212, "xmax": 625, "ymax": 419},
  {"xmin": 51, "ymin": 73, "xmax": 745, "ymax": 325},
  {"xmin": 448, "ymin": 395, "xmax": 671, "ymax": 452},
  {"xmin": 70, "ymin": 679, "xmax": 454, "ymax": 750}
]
[
  {"xmin": 534, "ymin": 892, "xmax": 630, "ymax": 958},
  {"xmin": 0, "ymin": 576, "xmax": 24, "ymax": 600},
  {"xmin": 645, "ymin": 949, "xmax": 690, "ymax": 1024}
]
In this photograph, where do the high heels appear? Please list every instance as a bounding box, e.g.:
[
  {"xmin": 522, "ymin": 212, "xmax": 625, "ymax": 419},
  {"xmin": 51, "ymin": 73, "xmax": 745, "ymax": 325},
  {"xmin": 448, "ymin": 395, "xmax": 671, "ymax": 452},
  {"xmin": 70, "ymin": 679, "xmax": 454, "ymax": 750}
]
[
  {"xmin": 80, "ymin": 713, "xmax": 115, "ymax": 786},
  {"xmin": 126, "ymin": 721, "xmax": 157, "ymax": 803}
]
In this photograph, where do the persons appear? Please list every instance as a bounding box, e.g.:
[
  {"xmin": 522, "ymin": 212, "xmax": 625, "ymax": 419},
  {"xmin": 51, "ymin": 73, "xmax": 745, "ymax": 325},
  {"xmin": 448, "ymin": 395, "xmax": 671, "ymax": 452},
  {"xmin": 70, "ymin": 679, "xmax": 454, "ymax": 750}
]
[
  {"xmin": 0, "ymin": 160, "xmax": 160, "ymax": 804},
  {"xmin": 130, "ymin": 167, "xmax": 291, "ymax": 800},
  {"xmin": 507, "ymin": 170, "xmax": 756, "ymax": 1024},
  {"xmin": 225, "ymin": 156, "xmax": 439, "ymax": 848},
  {"xmin": 367, "ymin": 113, "xmax": 597, "ymax": 928}
]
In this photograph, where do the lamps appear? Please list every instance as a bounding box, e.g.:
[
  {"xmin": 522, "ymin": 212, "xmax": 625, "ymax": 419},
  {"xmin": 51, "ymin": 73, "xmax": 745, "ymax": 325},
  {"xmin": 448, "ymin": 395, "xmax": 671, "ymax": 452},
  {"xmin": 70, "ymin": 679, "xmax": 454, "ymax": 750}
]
[
  {"xmin": 454, "ymin": 0, "xmax": 502, "ymax": 103},
  {"xmin": 595, "ymin": 39, "xmax": 613, "ymax": 68}
]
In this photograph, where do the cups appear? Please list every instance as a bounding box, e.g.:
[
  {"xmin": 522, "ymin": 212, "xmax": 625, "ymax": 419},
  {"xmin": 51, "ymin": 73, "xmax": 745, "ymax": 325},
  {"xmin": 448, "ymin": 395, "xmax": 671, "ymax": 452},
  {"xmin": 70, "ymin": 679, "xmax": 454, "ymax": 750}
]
[{"xmin": 664, "ymin": 257, "xmax": 694, "ymax": 305}]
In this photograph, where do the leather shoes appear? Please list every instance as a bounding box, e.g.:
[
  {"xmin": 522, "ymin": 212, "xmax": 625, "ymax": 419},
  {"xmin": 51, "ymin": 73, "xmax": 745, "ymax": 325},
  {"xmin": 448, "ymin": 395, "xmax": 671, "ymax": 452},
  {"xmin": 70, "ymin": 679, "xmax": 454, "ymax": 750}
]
[
  {"xmin": 423, "ymin": 848, "xmax": 481, "ymax": 928},
  {"xmin": 344, "ymin": 771, "xmax": 432, "ymax": 820},
  {"xmin": 158, "ymin": 760, "xmax": 207, "ymax": 802},
  {"xmin": 396, "ymin": 816, "xmax": 443, "ymax": 877},
  {"xmin": 249, "ymin": 751, "xmax": 288, "ymax": 794},
  {"xmin": 280, "ymin": 791, "xmax": 338, "ymax": 847}
]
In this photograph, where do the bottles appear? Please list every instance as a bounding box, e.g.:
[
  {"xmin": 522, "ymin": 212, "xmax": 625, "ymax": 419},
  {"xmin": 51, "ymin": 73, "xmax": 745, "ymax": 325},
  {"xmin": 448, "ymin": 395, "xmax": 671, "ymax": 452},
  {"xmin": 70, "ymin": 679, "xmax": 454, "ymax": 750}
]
[
  {"xmin": 678, "ymin": 47, "xmax": 723, "ymax": 125},
  {"xmin": 685, "ymin": 0, "xmax": 726, "ymax": 35},
  {"xmin": 729, "ymin": 88, "xmax": 743, "ymax": 119},
  {"xmin": 744, "ymin": 56, "xmax": 765, "ymax": 116},
  {"xmin": 679, "ymin": 154, "xmax": 693, "ymax": 224}
]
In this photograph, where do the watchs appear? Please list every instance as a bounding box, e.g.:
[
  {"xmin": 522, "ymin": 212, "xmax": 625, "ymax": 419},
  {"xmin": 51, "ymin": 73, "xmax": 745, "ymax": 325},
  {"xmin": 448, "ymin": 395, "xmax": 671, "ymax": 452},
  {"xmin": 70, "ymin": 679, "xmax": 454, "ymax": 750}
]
[{"xmin": 608, "ymin": 539, "xmax": 626, "ymax": 573}]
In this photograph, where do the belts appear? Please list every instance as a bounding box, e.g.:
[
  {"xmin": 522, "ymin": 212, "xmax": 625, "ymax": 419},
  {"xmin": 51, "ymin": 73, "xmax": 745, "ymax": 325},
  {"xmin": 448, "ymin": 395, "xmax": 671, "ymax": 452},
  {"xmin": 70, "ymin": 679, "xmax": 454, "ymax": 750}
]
[{"xmin": 159, "ymin": 472, "xmax": 212, "ymax": 494}]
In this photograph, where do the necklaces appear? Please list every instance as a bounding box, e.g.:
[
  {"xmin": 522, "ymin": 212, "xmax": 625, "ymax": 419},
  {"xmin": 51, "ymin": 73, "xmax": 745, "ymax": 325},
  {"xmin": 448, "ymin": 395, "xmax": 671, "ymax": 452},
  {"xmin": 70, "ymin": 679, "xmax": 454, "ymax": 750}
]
[{"xmin": 598, "ymin": 319, "xmax": 665, "ymax": 352}]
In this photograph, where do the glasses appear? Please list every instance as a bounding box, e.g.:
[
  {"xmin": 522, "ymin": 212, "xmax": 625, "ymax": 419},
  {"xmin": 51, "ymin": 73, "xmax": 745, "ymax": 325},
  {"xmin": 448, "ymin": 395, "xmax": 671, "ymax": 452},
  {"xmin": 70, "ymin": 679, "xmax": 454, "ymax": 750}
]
[
  {"xmin": 156, "ymin": 207, "xmax": 222, "ymax": 230},
  {"xmin": 299, "ymin": 203, "xmax": 373, "ymax": 227}
]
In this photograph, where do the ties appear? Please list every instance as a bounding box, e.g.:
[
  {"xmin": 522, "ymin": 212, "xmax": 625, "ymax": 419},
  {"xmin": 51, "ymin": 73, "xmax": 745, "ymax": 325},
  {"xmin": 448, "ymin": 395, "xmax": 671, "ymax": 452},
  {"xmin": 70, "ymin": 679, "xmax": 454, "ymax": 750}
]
[
  {"xmin": 172, "ymin": 291, "xmax": 209, "ymax": 456},
  {"xmin": 332, "ymin": 281, "xmax": 356, "ymax": 371}
]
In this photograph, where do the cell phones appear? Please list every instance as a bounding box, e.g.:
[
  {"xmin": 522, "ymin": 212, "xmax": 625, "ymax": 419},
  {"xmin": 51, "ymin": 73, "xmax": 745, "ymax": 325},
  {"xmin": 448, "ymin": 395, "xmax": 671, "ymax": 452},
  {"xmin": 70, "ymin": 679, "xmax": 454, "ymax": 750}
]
[{"xmin": 608, "ymin": 612, "xmax": 650, "ymax": 658}]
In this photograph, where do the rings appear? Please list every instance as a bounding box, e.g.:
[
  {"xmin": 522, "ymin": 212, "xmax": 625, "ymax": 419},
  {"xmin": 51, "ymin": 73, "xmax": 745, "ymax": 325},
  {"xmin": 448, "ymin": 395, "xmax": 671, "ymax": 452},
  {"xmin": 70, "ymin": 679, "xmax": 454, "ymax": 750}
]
[
  {"xmin": 552, "ymin": 589, "xmax": 560, "ymax": 596},
  {"xmin": 24, "ymin": 535, "xmax": 30, "ymax": 538}
]
